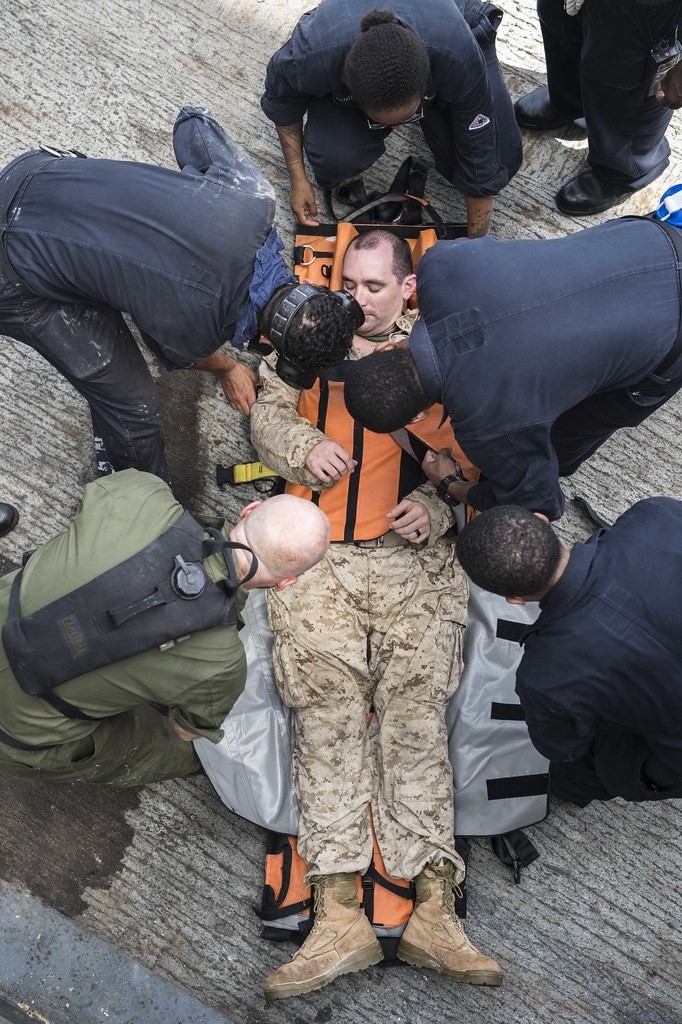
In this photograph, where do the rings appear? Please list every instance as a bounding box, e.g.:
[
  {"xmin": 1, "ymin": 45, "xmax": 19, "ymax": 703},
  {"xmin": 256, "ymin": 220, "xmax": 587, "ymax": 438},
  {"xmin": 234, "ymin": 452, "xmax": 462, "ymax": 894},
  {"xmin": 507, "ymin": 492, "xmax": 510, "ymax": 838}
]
[{"xmin": 416, "ymin": 530, "xmax": 420, "ymax": 537}]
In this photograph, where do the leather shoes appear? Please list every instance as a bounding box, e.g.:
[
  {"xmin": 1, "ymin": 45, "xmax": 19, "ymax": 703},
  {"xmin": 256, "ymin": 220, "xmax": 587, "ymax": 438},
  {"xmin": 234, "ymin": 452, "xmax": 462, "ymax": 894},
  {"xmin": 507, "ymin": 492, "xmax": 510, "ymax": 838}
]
[
  {"xmin": 513, "ymin": 84, "xmax": 586, "ymax": 130},
  {"xmin": 0, "ymin": 500, "xmax": 19, "ymax": 539},
  {"xmin": 555, "ymin": 142, "xmax": 670, "ymax": 217}
]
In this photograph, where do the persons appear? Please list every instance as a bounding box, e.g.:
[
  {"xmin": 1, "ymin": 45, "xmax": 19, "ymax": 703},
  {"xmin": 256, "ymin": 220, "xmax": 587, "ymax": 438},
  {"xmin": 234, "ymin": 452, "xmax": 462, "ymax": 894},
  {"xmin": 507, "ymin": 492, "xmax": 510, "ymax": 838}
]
[
  {"xmin": 0, "ymin": 109, "xmax": 364, "ymax": 483},
  {"xmin": 250, "ymin": 230, "xmax": 503, "ymax": 1000},
  {"xmin": 344, "ymin": 214, "xmax": 682, "ymax": 521},
  {"xmin": 514, "ymin": 0, "xmax": 682, "ymax": 215},
  {"xmin": 0, "ymin": 467, "xmax": 330, "ymax": 785},
  {"xmin": 457, "ymin": 496, "xmax": 682, "ymax": 808},
  {"xmin": 261, "ymin": 0, "xmax": 522, "ymax": 239}
]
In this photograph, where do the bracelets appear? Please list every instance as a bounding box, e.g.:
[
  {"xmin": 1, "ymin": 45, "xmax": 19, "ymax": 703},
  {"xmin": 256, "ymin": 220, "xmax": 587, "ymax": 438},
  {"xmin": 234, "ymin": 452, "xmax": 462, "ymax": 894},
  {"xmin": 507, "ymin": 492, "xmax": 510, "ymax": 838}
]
[{"xmin": 437, "ymin": 474, "xmax": 467, "ymax": 507}]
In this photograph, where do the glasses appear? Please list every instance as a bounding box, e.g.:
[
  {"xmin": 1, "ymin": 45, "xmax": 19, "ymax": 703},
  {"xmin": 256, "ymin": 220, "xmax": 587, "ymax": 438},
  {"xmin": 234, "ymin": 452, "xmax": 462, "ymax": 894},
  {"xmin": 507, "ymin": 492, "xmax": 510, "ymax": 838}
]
[{"xmin": 364, "ymin": 102, "xmax": 424, "ymax": 130}]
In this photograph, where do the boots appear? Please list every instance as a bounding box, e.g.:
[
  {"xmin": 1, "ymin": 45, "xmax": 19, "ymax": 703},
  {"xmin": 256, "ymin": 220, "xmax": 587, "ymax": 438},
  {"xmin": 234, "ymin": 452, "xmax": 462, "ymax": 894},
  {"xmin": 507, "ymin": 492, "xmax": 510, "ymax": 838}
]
[
  {"xmin": 263, "ymin": 871, "xmax": 384, "ymax": 1001},
  {"xmin": 397, "ymin": 861, "xmax": 503, "ymax": 987}
]
